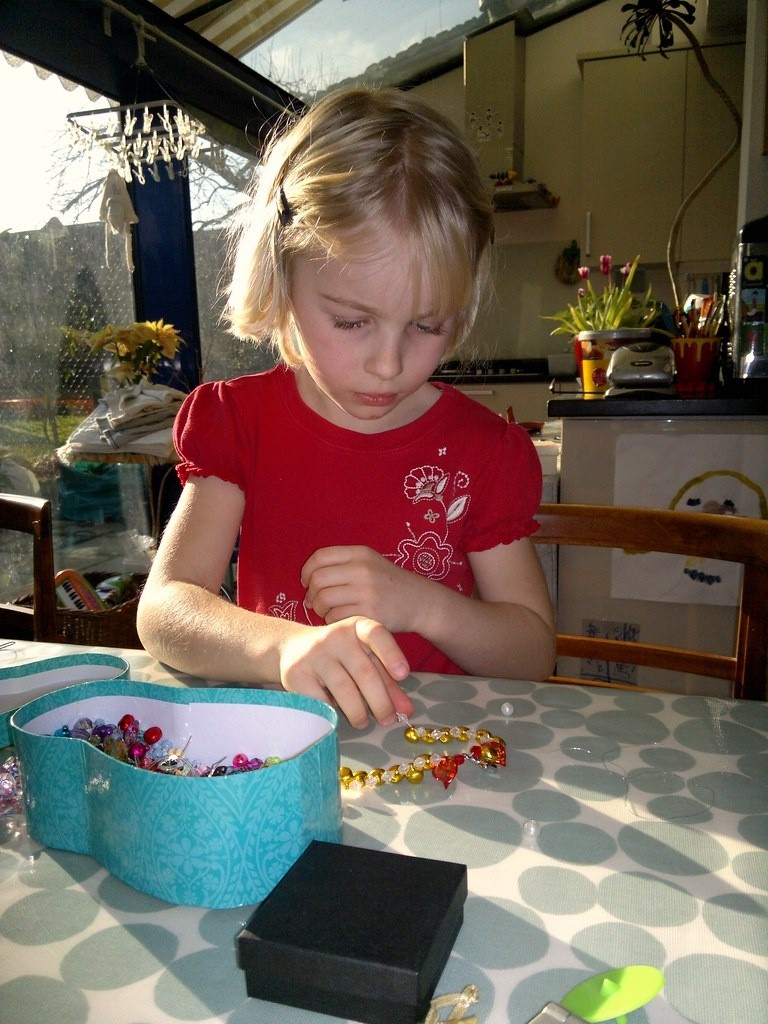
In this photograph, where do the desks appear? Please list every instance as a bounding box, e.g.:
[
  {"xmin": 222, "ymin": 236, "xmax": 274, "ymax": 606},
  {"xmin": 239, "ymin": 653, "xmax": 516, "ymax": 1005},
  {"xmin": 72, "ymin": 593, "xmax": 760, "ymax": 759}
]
[
  {"xmin": 0, "ymin": 637, "xmax": 768, "ymax": 1024},
  {"xmin": 56, "ymin": 447, "xmax": 184, "ymax": 465}
]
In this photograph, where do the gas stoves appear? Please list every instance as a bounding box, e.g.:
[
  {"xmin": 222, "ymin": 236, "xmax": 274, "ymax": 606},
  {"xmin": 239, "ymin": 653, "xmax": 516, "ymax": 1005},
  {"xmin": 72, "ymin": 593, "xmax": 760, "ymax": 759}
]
[{"xmin": 441, "ymin": 367, "xmax": 550, "ymax": 377}]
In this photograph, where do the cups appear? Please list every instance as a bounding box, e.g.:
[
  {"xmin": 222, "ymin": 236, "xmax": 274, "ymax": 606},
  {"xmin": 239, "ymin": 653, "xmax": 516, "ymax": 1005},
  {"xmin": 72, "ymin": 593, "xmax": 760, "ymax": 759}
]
[{"xmin": 671, "ymin": 338, "xmax": 722, "ymax": 396}]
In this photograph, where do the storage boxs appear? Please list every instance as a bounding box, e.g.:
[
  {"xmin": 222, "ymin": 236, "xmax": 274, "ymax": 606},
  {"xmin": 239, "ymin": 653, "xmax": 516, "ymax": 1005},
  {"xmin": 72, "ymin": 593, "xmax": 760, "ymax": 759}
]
[
  {"xmin": 234, "ymin": 839, "xmax": 467, "ymax": 1024},
  {"xmin": 9, "ymin": 678, "xmax": 337, "ymax": 909}
]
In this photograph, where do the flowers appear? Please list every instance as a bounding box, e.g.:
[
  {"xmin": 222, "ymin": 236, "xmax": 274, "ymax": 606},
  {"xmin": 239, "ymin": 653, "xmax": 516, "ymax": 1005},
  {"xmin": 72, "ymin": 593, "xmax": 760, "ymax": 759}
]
[
  {"xmin": 537, "ymin": 254, "xmax": 662, "ymax": 343},
  {"xmin": 88, "ymin": 318, "xmax": 187, "ymax": 387}
]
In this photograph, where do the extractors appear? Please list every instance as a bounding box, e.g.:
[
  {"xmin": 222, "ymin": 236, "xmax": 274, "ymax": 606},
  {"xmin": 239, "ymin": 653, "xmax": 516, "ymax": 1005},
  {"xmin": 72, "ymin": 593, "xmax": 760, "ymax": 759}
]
[{"xmin": 463, "ymin": 33, "xmax": 556, "ymax": 212}]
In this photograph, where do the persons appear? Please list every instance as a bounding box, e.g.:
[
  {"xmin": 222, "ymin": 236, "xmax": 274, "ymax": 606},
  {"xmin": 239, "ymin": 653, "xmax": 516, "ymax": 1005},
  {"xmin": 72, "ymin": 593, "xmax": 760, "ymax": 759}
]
[{"xmin": 137, "ymin": 87, "xmax": 557, "ymax": 731}]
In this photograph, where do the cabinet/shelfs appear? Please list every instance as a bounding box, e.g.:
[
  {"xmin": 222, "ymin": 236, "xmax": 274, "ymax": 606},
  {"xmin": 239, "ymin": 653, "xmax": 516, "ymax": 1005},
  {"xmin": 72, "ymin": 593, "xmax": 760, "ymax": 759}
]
[{"xmin": 577, "ymin": 45, "xmax": 740, "ymax": 272}]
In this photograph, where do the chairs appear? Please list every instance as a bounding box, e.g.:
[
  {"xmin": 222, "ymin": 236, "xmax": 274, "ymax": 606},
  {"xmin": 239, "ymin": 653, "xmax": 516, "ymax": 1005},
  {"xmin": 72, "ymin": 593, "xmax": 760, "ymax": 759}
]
[
  {"xmin": 528, "ymin": 503, "xmax": 768, "ymax": 699},
  {"xmin": 0, "ymin": 492, "xmax": 56, "ymax": 645}
]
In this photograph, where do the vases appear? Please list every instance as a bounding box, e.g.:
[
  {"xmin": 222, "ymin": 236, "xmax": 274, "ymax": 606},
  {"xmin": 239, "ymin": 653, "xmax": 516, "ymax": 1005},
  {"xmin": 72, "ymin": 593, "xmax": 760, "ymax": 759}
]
[{"xmin": 573, "ymin": 335, "xmax": 583, "ymax": 390}]
